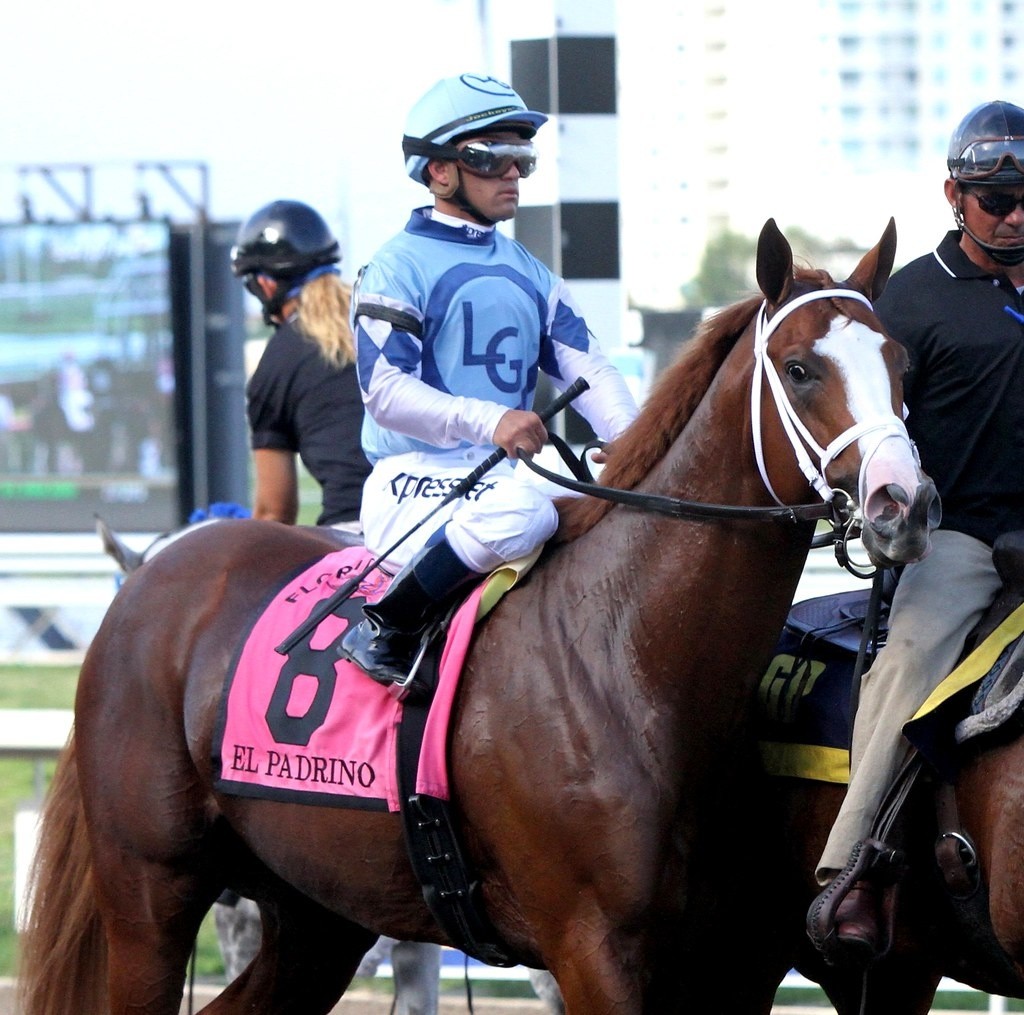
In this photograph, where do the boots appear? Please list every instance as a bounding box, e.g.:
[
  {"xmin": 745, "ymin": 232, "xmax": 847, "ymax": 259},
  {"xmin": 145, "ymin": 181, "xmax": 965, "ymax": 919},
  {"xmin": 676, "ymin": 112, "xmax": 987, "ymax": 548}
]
[{"xmin": 337, "ymin": 520, "xmax": 496, "ymax": 686}]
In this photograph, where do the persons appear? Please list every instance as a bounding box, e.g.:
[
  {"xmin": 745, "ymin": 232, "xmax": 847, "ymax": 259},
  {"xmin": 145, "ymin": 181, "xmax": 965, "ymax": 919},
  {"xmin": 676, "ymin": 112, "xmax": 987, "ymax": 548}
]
[
  {"xmin": 816, "ymin": 100, "xmax": 1024, "ymax": 948},
  {"xmin": 230, "ymin": 201, "xmax": 366, "ymax": 534},
  {"xmin": 350, "ymin": 76, "xmax": 643, "ymax": 694}
]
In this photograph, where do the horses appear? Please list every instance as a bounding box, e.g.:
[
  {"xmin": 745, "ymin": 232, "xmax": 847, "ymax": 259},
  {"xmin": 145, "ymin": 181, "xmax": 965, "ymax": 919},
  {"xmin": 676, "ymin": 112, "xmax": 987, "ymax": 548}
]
[{"xmin": 6, "ymin": 216, "xmax": 1022, "ymax": 1014}]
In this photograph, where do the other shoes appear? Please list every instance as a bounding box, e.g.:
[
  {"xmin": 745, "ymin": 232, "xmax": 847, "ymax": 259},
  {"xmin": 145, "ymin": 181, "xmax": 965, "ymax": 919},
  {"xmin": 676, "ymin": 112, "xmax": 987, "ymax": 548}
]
[{"xmin": 812, "ymin": 865, "xmax": 885, "ymax": 944}]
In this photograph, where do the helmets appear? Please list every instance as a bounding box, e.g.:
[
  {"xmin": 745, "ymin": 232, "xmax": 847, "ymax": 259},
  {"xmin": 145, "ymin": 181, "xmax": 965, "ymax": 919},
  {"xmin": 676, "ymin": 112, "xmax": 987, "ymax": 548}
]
[
  {"xmin": 406, "ymin": 73, "xmax": 547, "ymax": 180},
  {"xmin": 948, "ymin": 101, "xmax": 1024, "ymax": 188},
  {"xmin": 228, "ymin": 201, "xmax": 341, "ymax": 284}
]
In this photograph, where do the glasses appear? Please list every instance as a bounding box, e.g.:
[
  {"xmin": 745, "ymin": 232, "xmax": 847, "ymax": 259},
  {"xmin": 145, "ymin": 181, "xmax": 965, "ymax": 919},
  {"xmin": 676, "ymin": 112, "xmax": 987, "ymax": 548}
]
[
  {"xmin": 956, "ymin": 139, "xmax": 1021, "ymax": 180},
  {"xmin": 960, "ymin": 187, "xmax": 1023, "ymax": 218},
  {"xmin": 452, "ymin": 141, "xmax": 537, "ymax": 177},
  {"xmin": 242, "ymin": 274, "xmax": 261, "ymax": 292}
]
